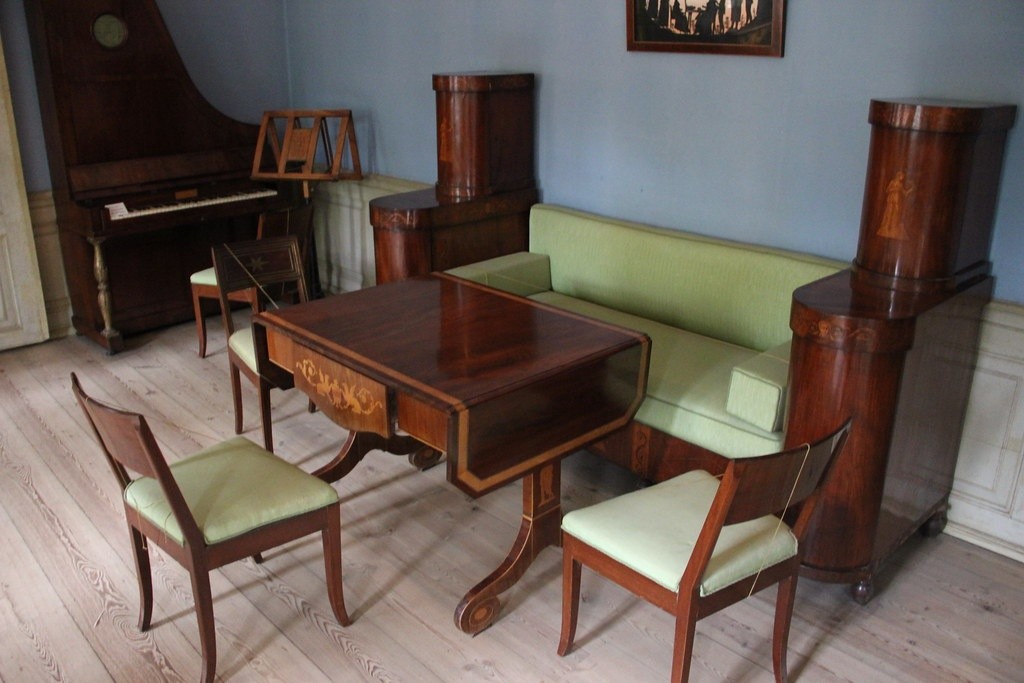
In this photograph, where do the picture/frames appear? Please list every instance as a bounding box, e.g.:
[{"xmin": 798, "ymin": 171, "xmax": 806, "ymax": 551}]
[{"xmin": 626, "ymin": 0, "xmax": 787, "ymax": 57}]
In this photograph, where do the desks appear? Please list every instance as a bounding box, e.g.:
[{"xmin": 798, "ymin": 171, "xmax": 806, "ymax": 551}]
[{"xmin": 250, "ymin": 270, "xmax": 653, "ymax": 634}]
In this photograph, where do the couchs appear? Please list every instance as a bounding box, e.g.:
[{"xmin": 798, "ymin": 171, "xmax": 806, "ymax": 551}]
[{"xmin": 440, "ymin": 204, "xmax": 848, "ymax": 484}]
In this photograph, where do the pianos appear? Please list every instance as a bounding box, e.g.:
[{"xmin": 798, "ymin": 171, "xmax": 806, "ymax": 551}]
[{"xmin": 44, "ymin": 145, "xmax": 303, "ymax": 357}]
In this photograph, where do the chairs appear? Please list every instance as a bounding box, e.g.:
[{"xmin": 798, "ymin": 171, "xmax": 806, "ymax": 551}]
[
  {"xmin": 557, "ymin": 418, "xmax": 854, "ymax": 683},
  {"xmin": 210, "ymin": 235, "xmax": 316, "ymax": 454},
  {"xmin": 190, "ymin": 198, "xmax": 320, "ymax": 359},
  {"xmin": 70, "ymin": 372, "xmax": 352, "ymax": 683}
]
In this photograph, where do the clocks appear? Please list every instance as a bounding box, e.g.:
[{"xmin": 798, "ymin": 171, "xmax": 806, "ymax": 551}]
[{"xmin": 89, "ymin": 12, "xmax": 129, "ymax": 52}]
